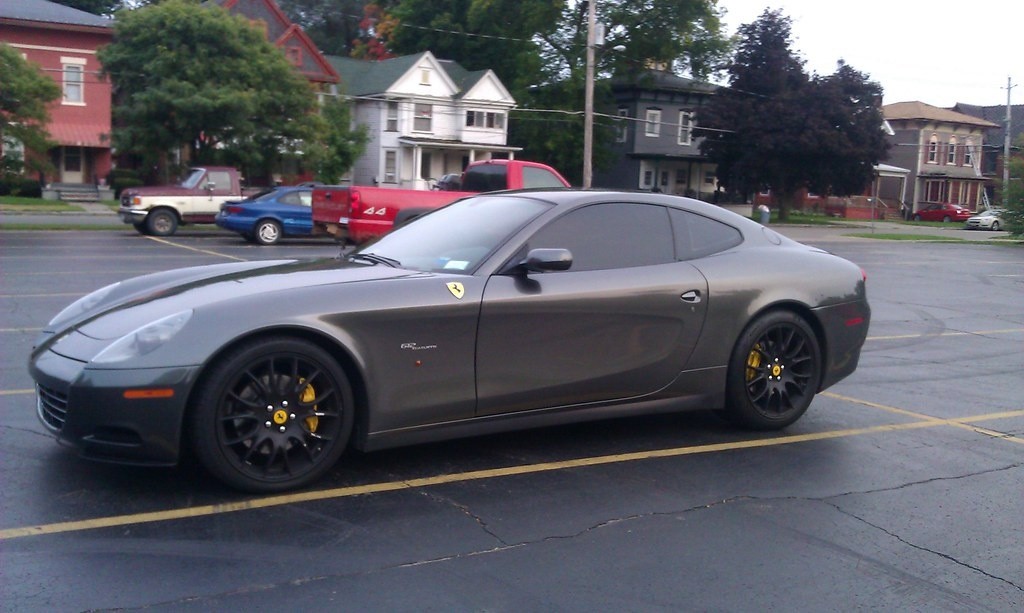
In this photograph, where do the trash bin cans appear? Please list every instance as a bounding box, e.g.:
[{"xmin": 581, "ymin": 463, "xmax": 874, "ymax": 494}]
[{"xmin": 758, "ymin": 204, "xmax": 770, "ymax": 223}]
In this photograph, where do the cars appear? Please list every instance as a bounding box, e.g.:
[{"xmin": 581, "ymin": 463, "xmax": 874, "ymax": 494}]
[
  {"xmin": 213, "ymin": 185, "xmax": 351, "ymax": 246},
  {"xmin": 967, "ymin": 209, "xmax": 1015, "ymax": 231},
  {"xmin": 912, "ymin": 202, "xmax": 972, "ymax": 223}
]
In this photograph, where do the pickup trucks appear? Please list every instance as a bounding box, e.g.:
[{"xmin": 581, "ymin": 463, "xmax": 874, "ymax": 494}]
[
  {"xmin": 312, "ymin": 158, "xmax": 575, "ymax": 250},
  {"xmin": 117, "ymin": 165, "xmax": 312, "ymax": 238}
]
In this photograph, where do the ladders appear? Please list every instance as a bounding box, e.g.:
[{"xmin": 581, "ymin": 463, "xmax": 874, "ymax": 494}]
[{"xmin": 969, "ymin": 146, "xmax": 990, "ymax": 211}]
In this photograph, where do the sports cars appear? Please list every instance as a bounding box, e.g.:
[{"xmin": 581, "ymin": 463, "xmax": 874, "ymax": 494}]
[{"xmin": 28, "ymin": 188, "xmax": 872, "ymax": 497}]
